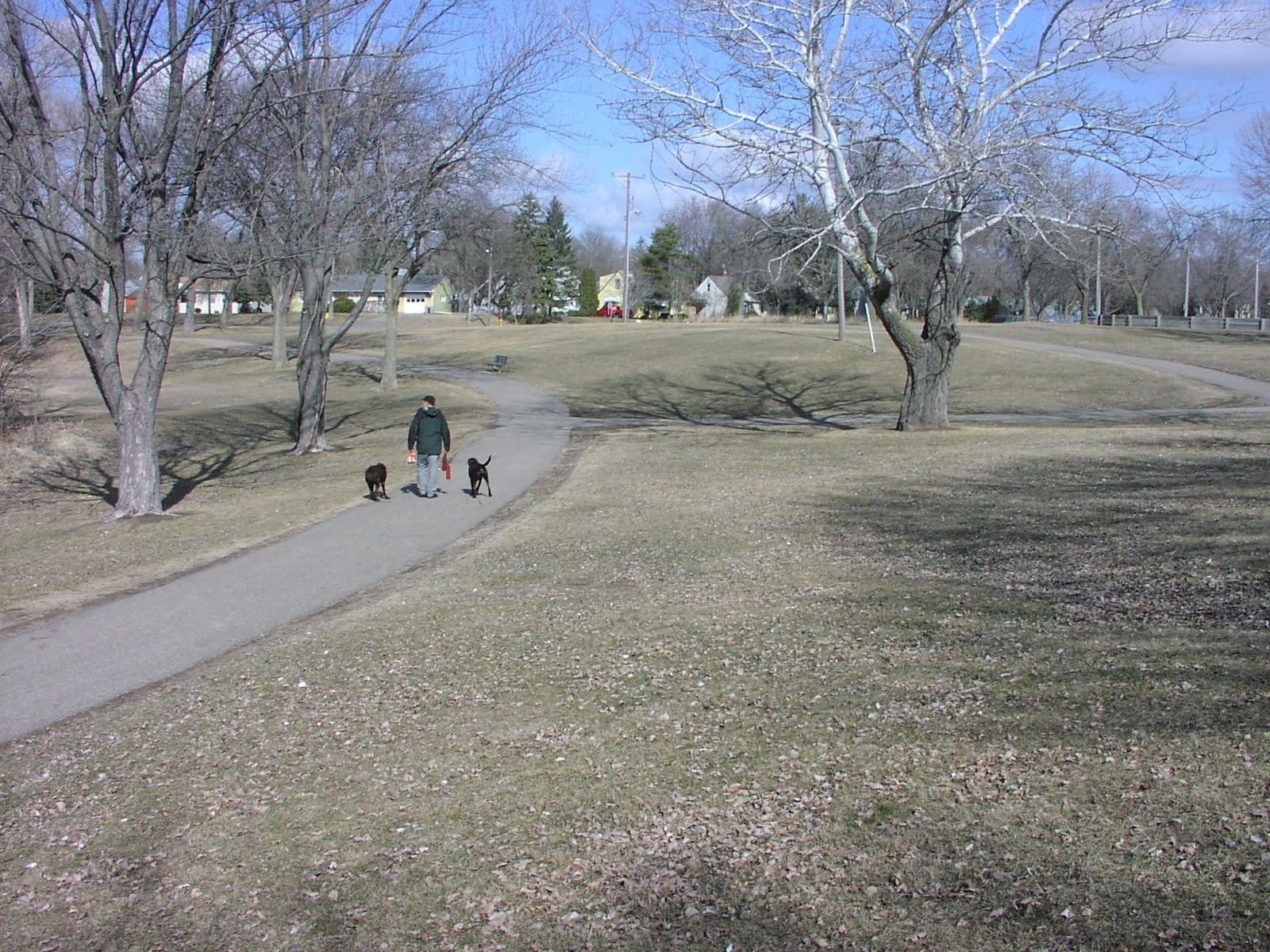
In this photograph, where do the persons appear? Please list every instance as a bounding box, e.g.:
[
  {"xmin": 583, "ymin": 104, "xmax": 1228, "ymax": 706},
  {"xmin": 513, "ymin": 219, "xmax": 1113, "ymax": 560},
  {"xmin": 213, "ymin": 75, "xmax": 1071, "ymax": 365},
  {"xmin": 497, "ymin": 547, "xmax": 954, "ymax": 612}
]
[{"xmin": 408, "ymin": 395, "xmax": 451, "ymax": 499}]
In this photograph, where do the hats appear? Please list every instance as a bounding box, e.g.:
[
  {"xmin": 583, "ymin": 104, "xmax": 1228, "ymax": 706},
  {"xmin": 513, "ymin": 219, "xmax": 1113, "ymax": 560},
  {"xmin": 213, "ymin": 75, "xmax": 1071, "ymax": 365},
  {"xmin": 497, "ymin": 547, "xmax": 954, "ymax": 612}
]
[{"xmin": 421, "ymin": 395, "xmax": 436, "ymax": 405}]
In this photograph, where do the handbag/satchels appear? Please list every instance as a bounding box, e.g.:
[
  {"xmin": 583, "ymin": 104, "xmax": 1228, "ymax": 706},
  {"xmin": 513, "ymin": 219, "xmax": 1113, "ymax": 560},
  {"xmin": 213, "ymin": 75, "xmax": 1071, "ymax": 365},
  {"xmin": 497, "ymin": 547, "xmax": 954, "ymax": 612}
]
[
  {"xmin": 445, "ymin": 464, "xmax": 451, "ymax": 480},
  {"xmin": 442, "ymin": 453, "xmax": 447, "ymax": 470},
  {"xmin": 406, "ymin": 451, "xmax": 416, "ymax": 463}
]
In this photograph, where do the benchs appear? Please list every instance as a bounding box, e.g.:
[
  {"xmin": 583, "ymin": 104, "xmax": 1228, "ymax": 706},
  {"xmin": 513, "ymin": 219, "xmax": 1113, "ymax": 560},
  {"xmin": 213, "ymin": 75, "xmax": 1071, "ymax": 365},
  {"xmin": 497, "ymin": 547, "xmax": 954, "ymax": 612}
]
[{"xmin": 487, "ymin": 356, "xmax": 507, "ymax": 372}]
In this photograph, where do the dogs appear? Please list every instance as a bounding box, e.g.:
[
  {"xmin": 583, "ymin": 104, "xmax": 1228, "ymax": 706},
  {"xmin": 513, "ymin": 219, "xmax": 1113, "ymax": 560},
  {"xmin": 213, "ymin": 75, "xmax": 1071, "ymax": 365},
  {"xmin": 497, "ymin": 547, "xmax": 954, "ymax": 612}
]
[
  {"xmin": 467, "ymin": 456, "xmax": 492, "ymax": 498},
  {"xmin": 364, "ymin": 463, "xmax": 391, "ymax": 501}
]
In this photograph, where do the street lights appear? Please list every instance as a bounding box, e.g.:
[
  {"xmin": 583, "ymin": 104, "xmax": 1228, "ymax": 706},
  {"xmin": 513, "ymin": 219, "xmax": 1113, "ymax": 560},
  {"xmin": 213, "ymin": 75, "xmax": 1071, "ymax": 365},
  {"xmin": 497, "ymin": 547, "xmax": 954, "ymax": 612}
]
[
  {"xmin": 621, "ymin": 209, "xmax": 643, "ymax": 321},
  {"xmin": 485, "ymin": 247, "xmax": 492, "ymax": 326}
]
